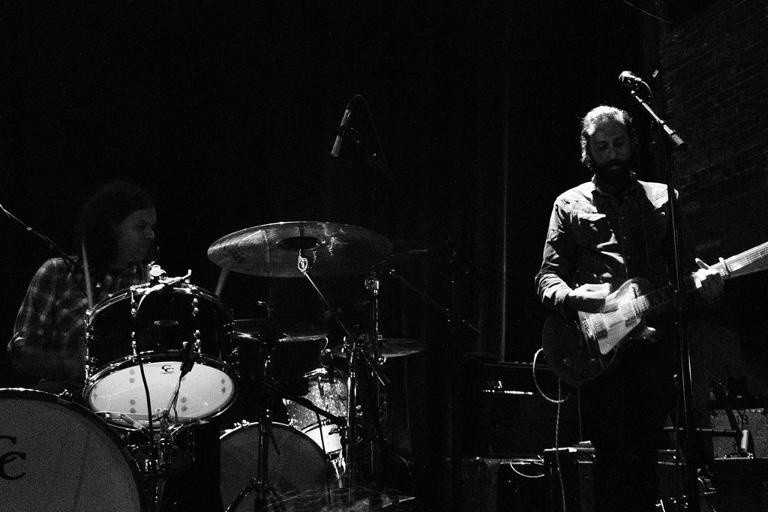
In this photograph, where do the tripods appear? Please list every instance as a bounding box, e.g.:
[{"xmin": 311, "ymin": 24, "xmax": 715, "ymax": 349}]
[{"xmin": 224, "ymin": 301, "xmax": 289, "ymax": 512}]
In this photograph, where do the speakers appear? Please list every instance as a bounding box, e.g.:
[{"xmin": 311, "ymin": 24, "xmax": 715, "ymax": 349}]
[
  {"xmin": 440, "ymin": 456, "xmax": 546, "ymax": 512},
  {"xmin": 442, "ymin": 363, "xmax": 580, "ymax": 460}
]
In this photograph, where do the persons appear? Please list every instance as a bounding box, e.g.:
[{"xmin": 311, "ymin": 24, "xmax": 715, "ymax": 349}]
[
  {"xmin": 4, "ymin": 181, "xmax": 178, "ymax": 406},
  {"xmin": 530, "ymin": 105, "xmax": 727, "ymax": 510}
]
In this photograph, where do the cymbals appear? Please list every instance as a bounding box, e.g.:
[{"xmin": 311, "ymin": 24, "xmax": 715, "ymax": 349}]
[
  {"xmin": 235, "ymin": 318, "xmax": 328, "ymax": 344},
  {"xmin": 335, "ymin": 337, "xmax": 429, "ymax": 359},
  {"xmin": 207, "ymin": 221, "xmax": 402, "ymax": 278}
]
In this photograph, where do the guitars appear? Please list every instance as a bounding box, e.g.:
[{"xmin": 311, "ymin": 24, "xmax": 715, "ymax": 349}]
[{"xmin": 544, "ymin": 242, "xmax": 768, "ymax": 388}]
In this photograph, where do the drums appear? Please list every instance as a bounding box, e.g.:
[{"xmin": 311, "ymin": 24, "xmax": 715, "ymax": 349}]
[
  {"xmin": 82, "ymin": 283, "xmax": 239, "ymax": 431},
  {"xmin": 218, "ymin": 418, "xmax": 337, "ymax": 512},
  {"xmin": 1, "ymin": 388, "xmax": 148, "ymax": 511},
  {"xmin": 281, "ymin": 366, "xmax": 368, "ymax": 456}
]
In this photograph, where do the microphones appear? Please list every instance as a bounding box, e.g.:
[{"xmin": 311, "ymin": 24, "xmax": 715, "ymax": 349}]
[
  {"xmin": 618, "ymin": 70, "xmax": 639, "ymax": 95},
  {"xmin": 330, "ymin": 108, "xmax": 353, "ymax": 159}
]
